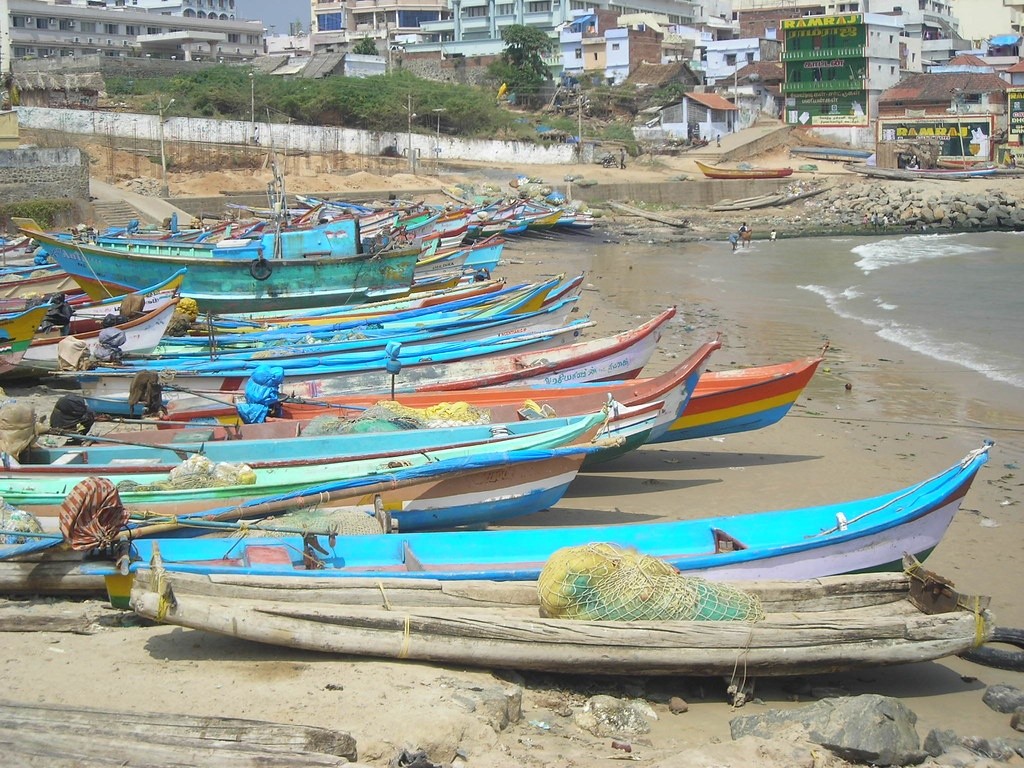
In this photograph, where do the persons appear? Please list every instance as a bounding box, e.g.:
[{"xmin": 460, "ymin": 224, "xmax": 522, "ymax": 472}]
[
  {"xmin": 702, "ymin": 136, "xmax": 707, "ymax": 142},
  {"xmin": 898, "ymin": 153, "xmax": 905, "ymax": 167},
  {"xmin": 729, "ymin": 232, "xmax": 741, "ymax": 250},
  {"xmin": 254, "ymin": 127, "xmax": 261, "ymax": 146},
  {"xmin": 717, "ymin": 134, "xmax": 721, "ymax": 147},
  {"xmin": 738, "ymin": 222, "xmax": 747, "ymax": 232},
  {"xmin": 769, "ymin": 229, "xmax": 776, "ymax": 241},
  {"xmin": 619, "ymin": 148, "xmax": 626, "ymax": 169}
]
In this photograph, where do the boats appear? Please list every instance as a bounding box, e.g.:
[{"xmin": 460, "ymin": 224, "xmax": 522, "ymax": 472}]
[
  {"xmin": 904, "ymin": 164, "xmax": 998, "ymax": 179},
  {"xmin": 791, "ymin": 146, "xmax": 872, "ymax": 163},
  {"xmin": 1, "ymin": 401, "xmax": 666, "ymax": 518},
  {"xmin": 156, "ymin": 337, "xmax": 828, "ymax": 444},
  {"xmin": 0, "ymin": 436, "xmax": 996, "ymax": 604},
  {"xmin": 694, "ymin": 159, "xmax": 794, "ymax": 179},
  {"xmin": 0, "ymin": 191, "xmax": 677, "ymax": 419},
  {"xmin": 106, "ymin": 540, "xmax": 997, "ymax": 677},
  {"xmin": 0, "ymin": 392, "xmax": 626, "ymax": 532}
]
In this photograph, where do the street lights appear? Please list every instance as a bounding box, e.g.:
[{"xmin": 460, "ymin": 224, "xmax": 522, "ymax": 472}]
[
  {"xmin": 408, "ymin": 113, "xmax": 416, "ymax": 169},
  {"xmin": 248, "ymin": 72, "xmax": 254, "ymax": 137},
  {"xmin": 159, "ymin": 99, "xmax": 176, "ymax": 175}
]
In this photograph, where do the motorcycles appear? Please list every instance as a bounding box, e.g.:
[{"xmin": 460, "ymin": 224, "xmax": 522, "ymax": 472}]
[{"xmin": 602, "ymin": 152, "xmax": 617, "ymax": 168}]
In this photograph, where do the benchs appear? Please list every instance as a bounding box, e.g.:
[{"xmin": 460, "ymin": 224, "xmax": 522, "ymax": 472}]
[
  {"xmin": 244, "ymin": 544, "xmax": 294, "ymax": 570},
  {"xmin": 171, "ymin": 430, "xmax": 215, "ymax": 443}
]
